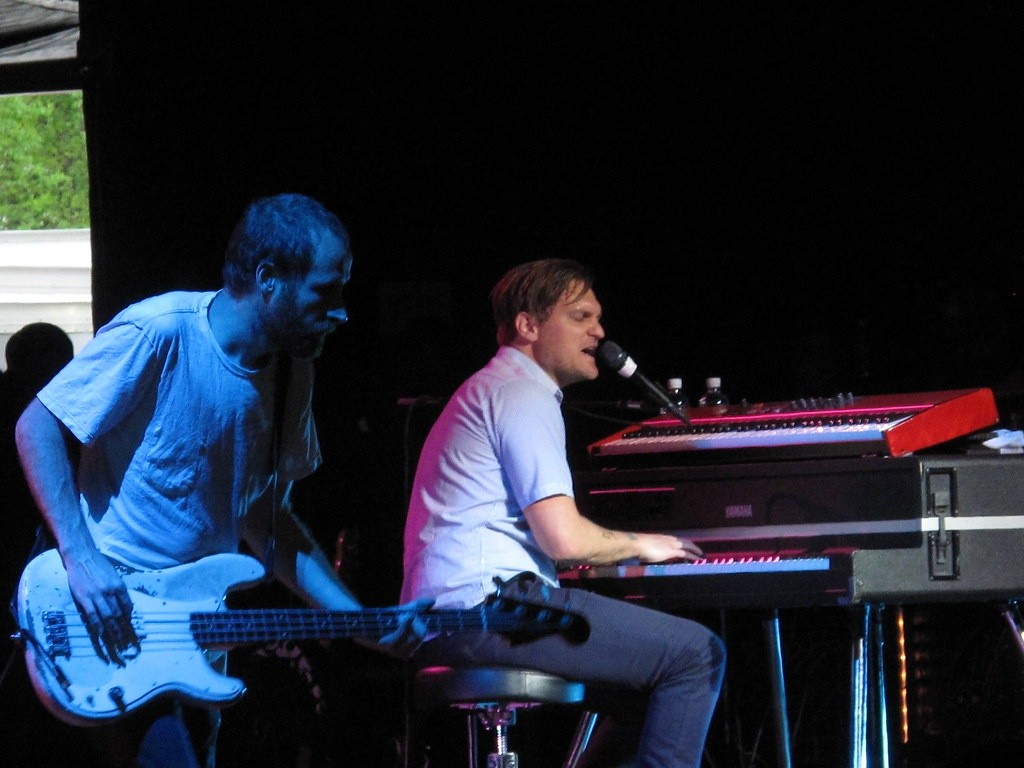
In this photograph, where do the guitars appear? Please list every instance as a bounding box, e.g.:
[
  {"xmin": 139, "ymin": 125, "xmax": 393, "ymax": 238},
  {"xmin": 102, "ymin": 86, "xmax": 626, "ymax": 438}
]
[{"xmin": 9, "ymin": 548, "xmax": 589, "ymax": 731}]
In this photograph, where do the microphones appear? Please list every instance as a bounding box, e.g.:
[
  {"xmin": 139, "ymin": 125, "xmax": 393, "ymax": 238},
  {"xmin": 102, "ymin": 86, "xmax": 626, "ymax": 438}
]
[
  {"xmin": 20, "ymin": 627, "xmax": 69, "ymax": 689},
  {"xmin": 598, "ymin": 339, "xmax": 692, "ymax": 427}
]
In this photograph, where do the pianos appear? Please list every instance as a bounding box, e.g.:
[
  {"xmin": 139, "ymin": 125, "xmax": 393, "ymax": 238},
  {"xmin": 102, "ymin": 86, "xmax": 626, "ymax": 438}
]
[{"xmin": 526, "ymin": 390, "xmax": 1024, "ymax": 768}]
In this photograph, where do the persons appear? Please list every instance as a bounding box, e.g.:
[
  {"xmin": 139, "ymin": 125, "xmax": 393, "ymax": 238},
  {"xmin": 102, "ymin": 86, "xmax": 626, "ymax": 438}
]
[
  {"xmin": 8, "ymin": 194, "xmax": 437, "ymax": 768},
  {"xmin": 0, "ymin": 318, "xmax": 86, "ymax": 573},
  {"xmin": 392, "ymin": 255, "xmax": 735, "ymax": 768}
]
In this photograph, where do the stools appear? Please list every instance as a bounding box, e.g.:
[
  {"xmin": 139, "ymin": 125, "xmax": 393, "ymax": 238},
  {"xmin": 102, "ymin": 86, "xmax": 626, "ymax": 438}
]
[{"xmin": 413, "ymin": 666, "xmax": 587, "ymax": 768}]
